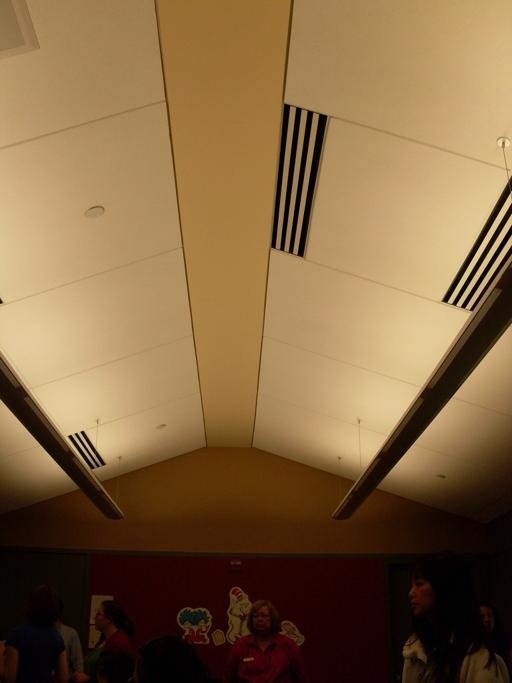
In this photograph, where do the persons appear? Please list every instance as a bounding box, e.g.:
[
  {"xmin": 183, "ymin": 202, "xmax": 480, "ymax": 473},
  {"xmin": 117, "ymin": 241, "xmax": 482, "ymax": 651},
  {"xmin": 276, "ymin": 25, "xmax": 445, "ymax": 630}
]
[
  {"xmin": 398, "ymin": 555, "xmax": 511, "ymax": 683},
  {"xmin": 219, "ymin": 599, "xmax": 310, "ymax": 682},
  {"xmin": 1, "ymin": 579, "xmax": 211, "ymax": 682}
]
